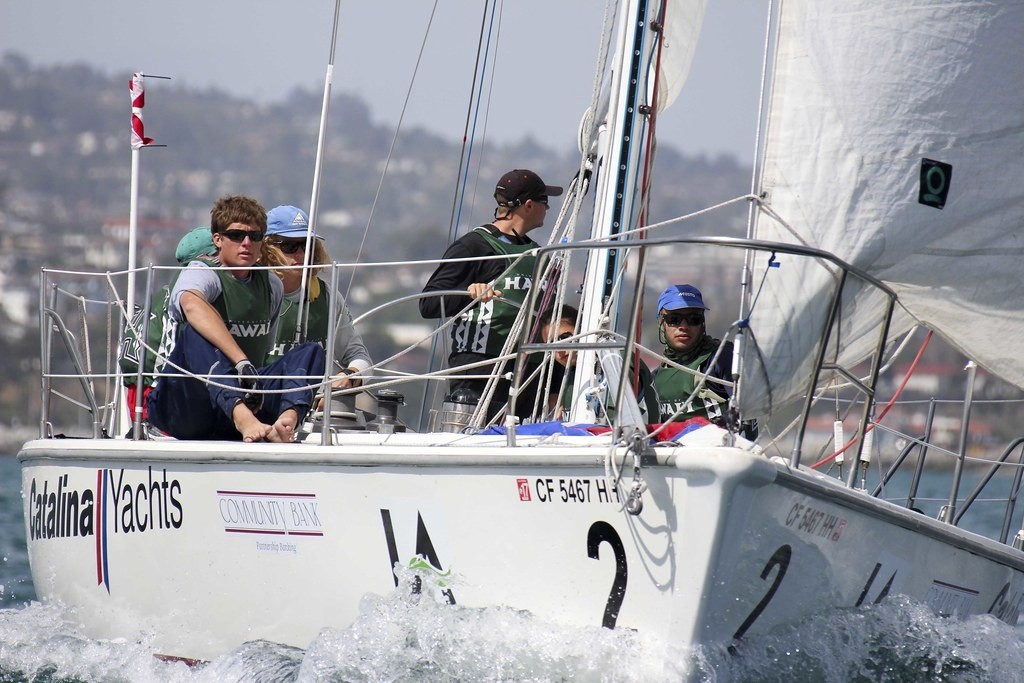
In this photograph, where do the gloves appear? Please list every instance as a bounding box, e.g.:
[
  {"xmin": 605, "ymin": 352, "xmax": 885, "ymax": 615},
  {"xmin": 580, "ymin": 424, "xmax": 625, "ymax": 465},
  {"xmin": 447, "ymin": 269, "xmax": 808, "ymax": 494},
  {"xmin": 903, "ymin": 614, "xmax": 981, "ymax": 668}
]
[{"xmin": 234, "ymin": 359, "xmax": 265, "ymax": 414}]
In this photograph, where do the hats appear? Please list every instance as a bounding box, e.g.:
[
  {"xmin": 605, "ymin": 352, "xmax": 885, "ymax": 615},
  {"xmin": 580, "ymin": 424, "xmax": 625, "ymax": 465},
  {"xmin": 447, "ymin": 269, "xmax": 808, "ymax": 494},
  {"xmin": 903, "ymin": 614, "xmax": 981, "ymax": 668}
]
[
  {"xmin": 494, "ymin": 169, "xmax": 563, "ymax": 206},
  {"xmin": 656, "ymin": 284, "xmax": 710, "ymax": 318},
  {"xmin": 264, "ymin": 204, "xmax": 325, "ymax": 240},
  {"xmin": 176, "ymin": 227, "xmax": 217, "ymax": 262}
]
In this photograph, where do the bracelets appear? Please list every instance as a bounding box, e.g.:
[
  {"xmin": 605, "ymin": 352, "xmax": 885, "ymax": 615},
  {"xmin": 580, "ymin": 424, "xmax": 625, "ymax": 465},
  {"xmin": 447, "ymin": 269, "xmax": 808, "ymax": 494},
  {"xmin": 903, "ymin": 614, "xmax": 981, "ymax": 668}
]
[{"xmin": 338, "ymin": 368, "xmax": 357, "ymax": 387}]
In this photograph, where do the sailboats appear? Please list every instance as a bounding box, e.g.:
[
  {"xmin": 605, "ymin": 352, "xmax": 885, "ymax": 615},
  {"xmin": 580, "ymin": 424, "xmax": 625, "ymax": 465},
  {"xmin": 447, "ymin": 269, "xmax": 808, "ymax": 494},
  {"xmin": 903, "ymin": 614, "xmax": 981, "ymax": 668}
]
[{"xmin": 15, "ymin": 1, "xmax": 1024, "ymax": 683}]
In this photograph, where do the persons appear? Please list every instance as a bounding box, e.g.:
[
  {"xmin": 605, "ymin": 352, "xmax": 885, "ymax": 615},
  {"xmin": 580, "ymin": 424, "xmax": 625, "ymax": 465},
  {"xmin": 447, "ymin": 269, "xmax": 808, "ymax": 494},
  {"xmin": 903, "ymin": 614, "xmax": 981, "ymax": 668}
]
[
  {"xmin": 118, "ymin": 226, "xmax": 219, "ymax": 438},
  {"xmin": 418, "ymin": 169, "xmax": 563, "ymax": 425},
  {"xmin": 538, "ymin": 304, "xmax": 660, "ymax": 424},
  {"xmin": 146, "ymin": 194, "xmax": 325, "ymax": 444},
  {"xmin": 653, "ymin": 284, "xmax": 758, "ymax": 441},
  {"xmin": 255, "ymin": 203, "xmax": 372, "ymax": 412}
]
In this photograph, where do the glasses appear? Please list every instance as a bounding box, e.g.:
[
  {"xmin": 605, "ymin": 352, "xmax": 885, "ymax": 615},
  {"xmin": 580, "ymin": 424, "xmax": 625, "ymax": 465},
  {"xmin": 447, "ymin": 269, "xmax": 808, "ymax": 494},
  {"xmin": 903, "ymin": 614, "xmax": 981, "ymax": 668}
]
[
  {"xmin": 531, "ymin": 196, "xmax": 548, "ymax": 204},
  {"xmin": 550, "ymin": 332, "xmax": 573, "ymax": 342},
  {"xmin": 218, "ymin": 229, "xmax": 263, "ymax": 241},
  {"xmin": 272, "ymin": 241, "xmax": 312, "ymax": 254},
  {"xmin": 663, "ymin": 312, "xmax": 704, "ymax": 326}
]
